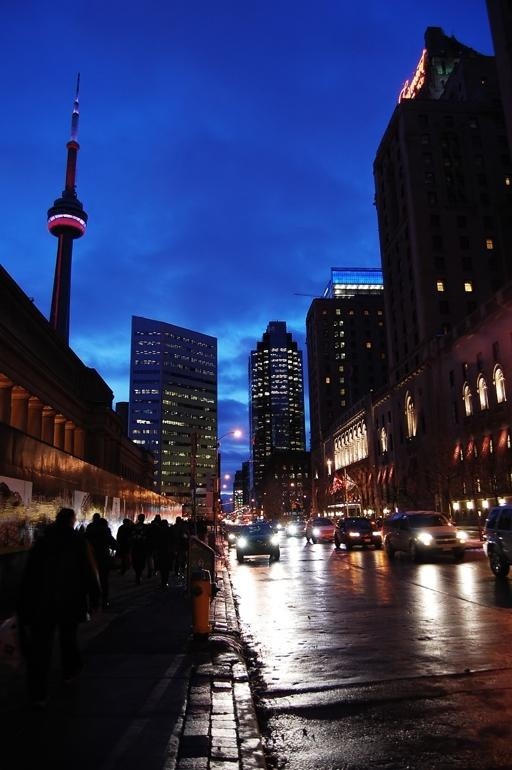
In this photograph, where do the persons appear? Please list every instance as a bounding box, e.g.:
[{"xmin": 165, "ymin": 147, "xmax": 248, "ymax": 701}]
[{"xmin": 14, "ymin": 505, "xmax": 218, "ymax": 694}]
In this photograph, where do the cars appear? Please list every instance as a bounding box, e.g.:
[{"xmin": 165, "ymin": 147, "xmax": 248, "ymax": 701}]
[{"xmin": 218, "ymin": 508, "xmax": 470, "ymax": 566}]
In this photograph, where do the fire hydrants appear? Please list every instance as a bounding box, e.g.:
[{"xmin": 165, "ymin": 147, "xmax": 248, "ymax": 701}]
[{"xmin": 187, "ymin": 564, "xmax": 220, "ymax": 643}]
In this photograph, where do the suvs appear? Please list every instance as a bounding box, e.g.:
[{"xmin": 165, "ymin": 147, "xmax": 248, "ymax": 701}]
[{"xmin": 479, "ymin": 503, "xmax": 512, "ymax": 579}]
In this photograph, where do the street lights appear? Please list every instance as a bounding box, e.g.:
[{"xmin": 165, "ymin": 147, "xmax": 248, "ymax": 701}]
[
  {"xmin": 249, "ymin": 498, "xmax": 256, "ymax": 509},
  {"xmin": 214, "ymin": 430, "xmax": 242, "ymax": 513}
]
